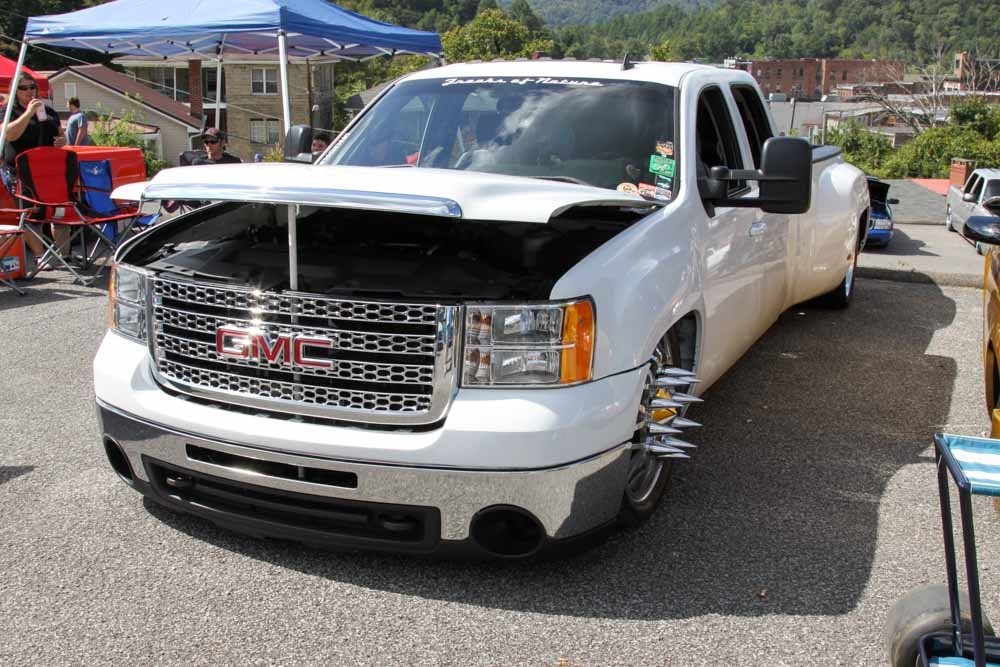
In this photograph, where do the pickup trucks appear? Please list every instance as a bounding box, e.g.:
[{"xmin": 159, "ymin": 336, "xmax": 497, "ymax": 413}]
[
  {"xmin": 945, "ymin": 167, "xmax": 1000, "ymax": 256},
  {"xmin": 92, "ymin": 54, "xmax": 872, "ymax": 568}
]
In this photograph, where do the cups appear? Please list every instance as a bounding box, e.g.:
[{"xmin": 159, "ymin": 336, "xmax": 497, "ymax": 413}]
[{"xmin": 35, "ymin": 101, "xmax": 47, "ymax": 122}]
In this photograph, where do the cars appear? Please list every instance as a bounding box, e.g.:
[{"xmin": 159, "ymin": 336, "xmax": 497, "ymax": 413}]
[
  {"xmin": 962, "ymin": 195, "xmax": 1000, "ymax": 440},
  {"xmin": 863, "ymin": 176, "xmax": 900, "ymax": 250}
]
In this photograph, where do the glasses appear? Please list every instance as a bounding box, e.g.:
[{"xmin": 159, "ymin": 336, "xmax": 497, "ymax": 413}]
[
  {"xmin": 203, "ymin": 139, "xmax": 220, "ymax": 145},
  {"xmin": 17, "ymin": 84, "xmax": 36, "ymax": 91}
]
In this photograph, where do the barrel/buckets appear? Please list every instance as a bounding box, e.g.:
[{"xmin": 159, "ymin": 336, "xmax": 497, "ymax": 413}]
[{"xmin": 0, "ymin": 225, "xmax": 25, "ymax": 280}]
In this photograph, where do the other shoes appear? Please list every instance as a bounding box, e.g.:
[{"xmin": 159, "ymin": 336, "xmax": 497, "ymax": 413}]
[
  {"xmin": 36, "ymin": 257, "xmax": 53, "ymax": 271},
  {"xmin": 57, "ymin": 256, "xmax": 76, "ymax": 266}
]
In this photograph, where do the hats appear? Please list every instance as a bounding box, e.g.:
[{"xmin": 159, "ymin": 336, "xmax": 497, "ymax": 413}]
[{"xmin": 201, "ymin": 127, "xmax": 225, "ymax": 140}]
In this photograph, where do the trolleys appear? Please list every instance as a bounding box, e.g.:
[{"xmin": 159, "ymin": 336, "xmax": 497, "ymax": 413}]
[{"xmin": 885, "ymin": 432, "xmax": 1000, "ymax": 667}]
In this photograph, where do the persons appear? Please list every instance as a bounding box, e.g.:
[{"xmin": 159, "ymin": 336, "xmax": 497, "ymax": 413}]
[
  {"xmin": 66, "ymin": 96, "xmax": 88, "ymax": 146},
  {"xmin": 0, "ymin": 71, "xmax": 89, "ymax": 270},
  {"xmin": 192, "ymin": 128, "xmax": 241, "ymax": 165},
  {"xmin": 312, "ymin": 133, "xmax": 330, "ymax": 151}
]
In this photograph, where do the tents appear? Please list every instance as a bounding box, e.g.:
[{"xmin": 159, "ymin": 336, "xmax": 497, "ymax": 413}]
[
  {"xmin": 0, "ymin": 0, "xmax": 447, "ymax": 164},
  {"xmin": 0, "ymin": 55, "xmax": 54, "ymax": 109}
]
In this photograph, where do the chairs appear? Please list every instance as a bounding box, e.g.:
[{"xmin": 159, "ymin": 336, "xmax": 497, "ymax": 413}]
[
  {"xmin": 177, "ymin": 148, "xmax": 217, "ymax": 213},
  {"xmin": 14, "ymin": 147, "xmax": 145, "ymax": 287},
  {"xmin": 1, "ymin": 177, "xmax": 41, "ymax": 295},
  {"xmin": 81, "ymin": 160, "xmax": 162, "ymax": 269}
]
